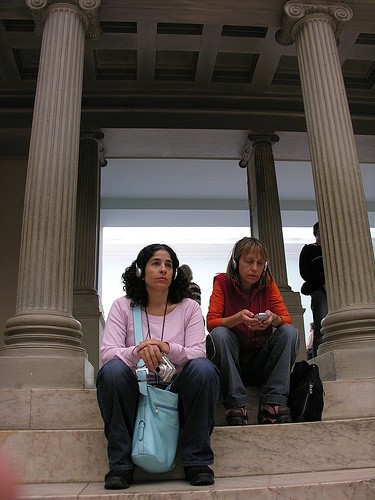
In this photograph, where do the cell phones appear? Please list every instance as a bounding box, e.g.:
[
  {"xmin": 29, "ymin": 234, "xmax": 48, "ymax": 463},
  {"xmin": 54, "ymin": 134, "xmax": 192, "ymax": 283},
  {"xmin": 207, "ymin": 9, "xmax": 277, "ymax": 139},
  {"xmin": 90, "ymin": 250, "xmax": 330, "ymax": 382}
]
[{"xmin": 255, "ymin": 313, "xmax": 268, "ymax": 321}]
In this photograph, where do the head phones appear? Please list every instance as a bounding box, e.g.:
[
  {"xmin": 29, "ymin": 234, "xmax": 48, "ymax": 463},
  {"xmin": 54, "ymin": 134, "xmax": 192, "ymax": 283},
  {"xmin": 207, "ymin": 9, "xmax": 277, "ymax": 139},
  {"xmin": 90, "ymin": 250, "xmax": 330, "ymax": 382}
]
[
  {"xmin": 135, "ymin": 262, "xmax": 178, "ymax": 280},
  {"xmin": 231, "ymin": 242, "xmax": 268, "ymax": 272}
]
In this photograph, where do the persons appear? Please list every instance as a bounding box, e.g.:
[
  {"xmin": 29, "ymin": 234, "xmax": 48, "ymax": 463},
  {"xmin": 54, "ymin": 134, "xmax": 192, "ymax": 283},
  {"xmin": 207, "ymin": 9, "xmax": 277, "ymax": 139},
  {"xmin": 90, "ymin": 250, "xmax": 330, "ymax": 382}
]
[
  {"xmin": 94, "ymin": 243, "xmax": 219, "ymax": 490},
  {"xmin": 176, "ymin": 266, "xmax": 202, "ymax": 306},
  {"xmin": 206, "ymin": 237, "xmax": 300, "ymax": 426},
  {"xmin": 299, "ymin": 221, "xmax": 328, "ymax": 356}
]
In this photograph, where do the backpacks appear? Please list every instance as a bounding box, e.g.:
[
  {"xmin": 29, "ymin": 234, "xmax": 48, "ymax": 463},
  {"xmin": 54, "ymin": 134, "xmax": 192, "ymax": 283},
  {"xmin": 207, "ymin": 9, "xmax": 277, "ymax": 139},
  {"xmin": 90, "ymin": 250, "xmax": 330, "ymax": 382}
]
[{"xmin": 289, "ymin": 360, "xmax": 326, "ymax": 423}]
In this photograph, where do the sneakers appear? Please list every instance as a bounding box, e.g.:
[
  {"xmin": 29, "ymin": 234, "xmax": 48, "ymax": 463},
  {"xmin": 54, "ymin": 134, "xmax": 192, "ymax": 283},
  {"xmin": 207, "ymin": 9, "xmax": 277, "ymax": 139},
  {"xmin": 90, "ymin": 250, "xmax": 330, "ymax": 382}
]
[
  {"xmin": 258, "ymin": 416, "xmax": 291, "ymax": 423},
  {"xmin": 225, "ymin": 405, "xmax": 248, "ymax": 417},
  {"xmin": 183, "ymin": 464, "xmax": 214, "ymax": 486},
  {"xmin": 257, "ymin": 403, "xmax": 290, "ymax": 416},
  {"xmin": 225, "ymin": 417, "xmax": 247, "ymax": 425},
  {"xmin": 105, "ymin": 474, "xmax": 133, "ymax": 489}
]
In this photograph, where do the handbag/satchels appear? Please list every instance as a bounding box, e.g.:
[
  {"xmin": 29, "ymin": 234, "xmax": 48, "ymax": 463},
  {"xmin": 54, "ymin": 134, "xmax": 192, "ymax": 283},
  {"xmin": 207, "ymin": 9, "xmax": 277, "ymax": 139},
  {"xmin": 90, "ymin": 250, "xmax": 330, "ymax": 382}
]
[
  {"xmin": 301, "ymin": 281, "xmax": 314, "ymax": 294},
  {"xmin": 131, "ymin": 368, "xmax": 181, "ymax": 474}
]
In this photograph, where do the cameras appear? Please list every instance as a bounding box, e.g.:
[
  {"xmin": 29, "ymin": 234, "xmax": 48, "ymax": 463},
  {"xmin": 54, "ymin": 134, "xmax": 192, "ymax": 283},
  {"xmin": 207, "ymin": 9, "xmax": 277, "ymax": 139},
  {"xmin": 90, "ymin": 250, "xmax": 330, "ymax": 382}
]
[{"xmin": 155, "ymin": 356, "xmax": 176, "ymax": 383}]
